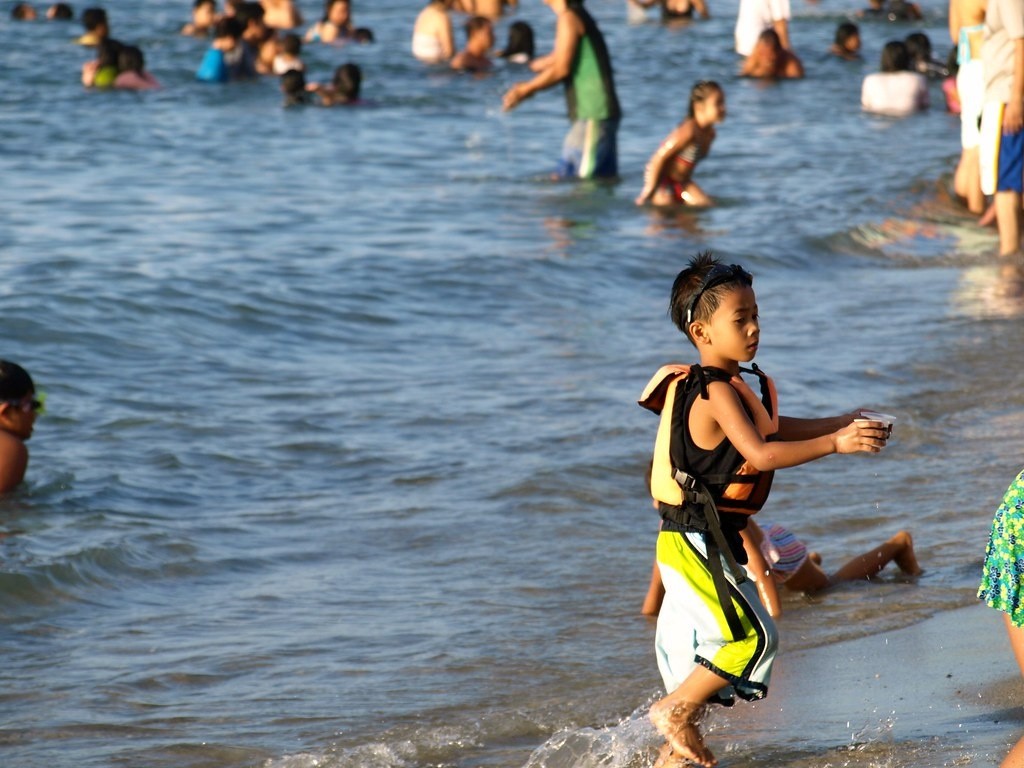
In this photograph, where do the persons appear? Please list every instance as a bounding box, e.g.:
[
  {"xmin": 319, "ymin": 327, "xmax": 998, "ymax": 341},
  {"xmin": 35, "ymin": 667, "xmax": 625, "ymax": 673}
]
[
  {"xmin": 12, "ymin": 0, "xmax": 374, "ymax": 108},
  {"xmin": 411, "ymin": 0, "xmax": 1024, "ymax": 254},
  {"xmin": 638, "ymin": 251, "xmax": 921, "ymax": 768},
  {"xmin": 977, "ymin": 470, "xmax": 1024, "ymax": 768},
  {"xmin": 0, "ymin": 360, "xmax": 37, "ymax": 491}
]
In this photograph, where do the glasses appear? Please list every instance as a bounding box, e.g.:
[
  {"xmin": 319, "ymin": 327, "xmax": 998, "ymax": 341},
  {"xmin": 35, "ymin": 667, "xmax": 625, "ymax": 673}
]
[
  {"xmin": 681, "ymin": 264, "xmax": 753, "ymax": 332},
  {"xmin": 10, "ymin": 400, "xmax": 41, "ymax": 410}
]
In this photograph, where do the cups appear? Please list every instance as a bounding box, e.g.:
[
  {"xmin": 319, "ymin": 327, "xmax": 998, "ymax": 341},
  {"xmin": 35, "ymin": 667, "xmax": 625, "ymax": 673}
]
[{"xmin": 860, "ymin": 413, "xmax": 895, "ymax": 449}]
